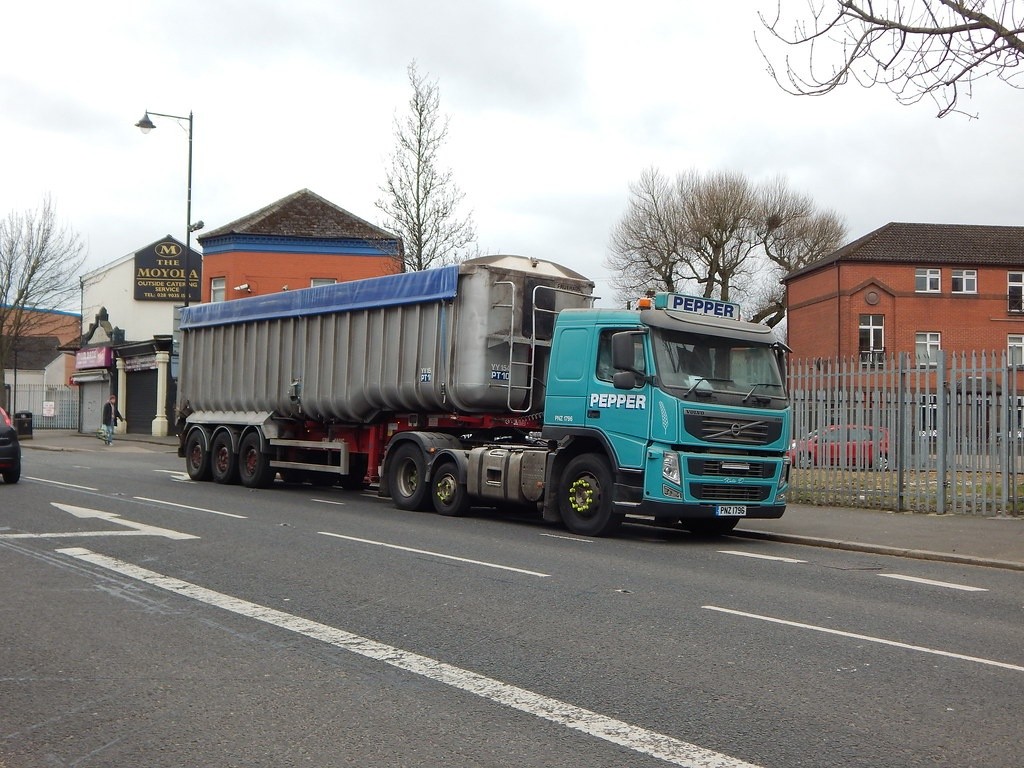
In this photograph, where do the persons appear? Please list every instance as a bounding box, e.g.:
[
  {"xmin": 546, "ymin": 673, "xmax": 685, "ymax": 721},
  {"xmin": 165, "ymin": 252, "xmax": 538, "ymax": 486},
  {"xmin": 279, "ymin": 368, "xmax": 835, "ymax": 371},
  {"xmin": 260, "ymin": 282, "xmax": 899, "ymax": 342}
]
[{"xmin": 103, "ymin": 395, "xmax": 124, "ymax": 447}]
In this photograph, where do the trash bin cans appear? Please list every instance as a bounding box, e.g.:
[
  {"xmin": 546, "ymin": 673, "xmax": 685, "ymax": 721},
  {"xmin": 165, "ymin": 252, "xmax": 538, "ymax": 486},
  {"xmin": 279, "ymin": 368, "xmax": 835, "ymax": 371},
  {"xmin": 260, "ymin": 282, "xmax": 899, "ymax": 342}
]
[{"xmin": 13, "ymin": 409, "xmax": 34, "ymax": 442}]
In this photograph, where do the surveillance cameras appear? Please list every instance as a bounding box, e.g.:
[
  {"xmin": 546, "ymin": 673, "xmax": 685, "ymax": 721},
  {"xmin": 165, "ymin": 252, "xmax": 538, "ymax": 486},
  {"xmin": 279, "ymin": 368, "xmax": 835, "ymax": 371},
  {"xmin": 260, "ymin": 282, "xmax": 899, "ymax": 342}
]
[
  {"xmin": 233, "ymin": 284, "xmax": 248, "ymax": 291},
  {"xmin": 283, "ymin": 285, "xmax": 288, "ymax": 291}
]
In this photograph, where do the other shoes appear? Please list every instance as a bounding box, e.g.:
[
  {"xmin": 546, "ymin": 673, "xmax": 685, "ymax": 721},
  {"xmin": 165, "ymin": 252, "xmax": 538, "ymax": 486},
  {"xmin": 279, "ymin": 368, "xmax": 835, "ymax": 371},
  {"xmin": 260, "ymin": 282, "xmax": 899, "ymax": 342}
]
[{"xmin": 105, "ymin": 440, "xmax": 114, "ymax": 446}]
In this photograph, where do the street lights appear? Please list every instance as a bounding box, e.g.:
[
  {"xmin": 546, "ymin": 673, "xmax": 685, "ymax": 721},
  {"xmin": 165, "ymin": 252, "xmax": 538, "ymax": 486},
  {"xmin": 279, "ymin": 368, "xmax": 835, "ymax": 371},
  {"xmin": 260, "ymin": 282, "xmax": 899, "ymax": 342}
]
[{"xmin": 135, "ymin": 109, "xmax": 206, "ymax": 307}]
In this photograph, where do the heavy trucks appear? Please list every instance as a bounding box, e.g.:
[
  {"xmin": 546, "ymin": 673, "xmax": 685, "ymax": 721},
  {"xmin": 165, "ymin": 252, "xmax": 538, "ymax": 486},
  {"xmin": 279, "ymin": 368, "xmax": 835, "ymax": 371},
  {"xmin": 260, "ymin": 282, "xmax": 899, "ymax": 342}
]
[{"xmin": 174, "ymin": 255, "xmax": 796, "ymax": 537}]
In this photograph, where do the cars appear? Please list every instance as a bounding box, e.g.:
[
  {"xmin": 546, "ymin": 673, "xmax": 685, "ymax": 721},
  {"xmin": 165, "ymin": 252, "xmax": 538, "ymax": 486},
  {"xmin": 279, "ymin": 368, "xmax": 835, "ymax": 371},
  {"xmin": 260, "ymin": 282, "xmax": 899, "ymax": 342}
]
[
  {"xmin": 0, "ymin": 408, "xmax": 21, "ymax": 483},
  {"xmin": 786, "ymin": 424, "xmax": 889, "ymax": 471}
]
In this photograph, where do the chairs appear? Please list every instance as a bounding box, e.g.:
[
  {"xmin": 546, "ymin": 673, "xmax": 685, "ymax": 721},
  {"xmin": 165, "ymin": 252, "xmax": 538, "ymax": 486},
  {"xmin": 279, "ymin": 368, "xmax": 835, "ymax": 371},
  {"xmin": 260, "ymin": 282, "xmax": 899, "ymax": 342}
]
[{"xmin": 677, "ymin": 346, "xmax": 735, "ymax": 388}]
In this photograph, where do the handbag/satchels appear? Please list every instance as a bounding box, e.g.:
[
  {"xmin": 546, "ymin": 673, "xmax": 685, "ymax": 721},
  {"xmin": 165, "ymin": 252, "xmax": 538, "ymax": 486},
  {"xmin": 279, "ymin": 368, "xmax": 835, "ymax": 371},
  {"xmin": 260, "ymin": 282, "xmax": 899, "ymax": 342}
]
[{"xmin": 97, "ymin": 424, "xmax": 111, "ymax": 441}]
